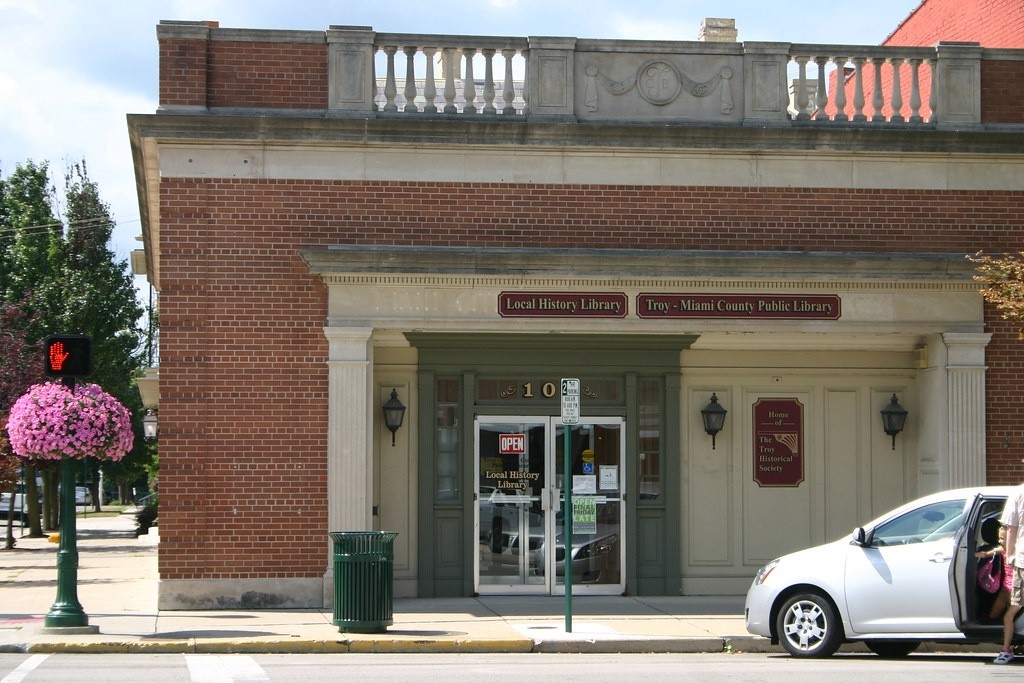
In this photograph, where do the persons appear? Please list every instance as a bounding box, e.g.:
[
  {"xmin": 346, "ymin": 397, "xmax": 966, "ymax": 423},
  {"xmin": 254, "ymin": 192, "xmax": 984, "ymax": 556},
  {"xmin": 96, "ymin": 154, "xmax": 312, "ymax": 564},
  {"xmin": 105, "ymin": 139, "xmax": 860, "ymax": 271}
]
[{"xmin": 976, "ymin": 459, "xmax": 1024, "ymax": 665}]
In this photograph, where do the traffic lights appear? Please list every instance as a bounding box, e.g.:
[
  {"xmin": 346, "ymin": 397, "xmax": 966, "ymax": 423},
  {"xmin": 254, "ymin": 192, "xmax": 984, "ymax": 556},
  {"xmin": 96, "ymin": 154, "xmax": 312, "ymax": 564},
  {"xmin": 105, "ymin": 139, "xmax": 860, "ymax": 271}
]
[{"xmin": 45, "ymin": 336, "xmax": 92, "ymax": 377}]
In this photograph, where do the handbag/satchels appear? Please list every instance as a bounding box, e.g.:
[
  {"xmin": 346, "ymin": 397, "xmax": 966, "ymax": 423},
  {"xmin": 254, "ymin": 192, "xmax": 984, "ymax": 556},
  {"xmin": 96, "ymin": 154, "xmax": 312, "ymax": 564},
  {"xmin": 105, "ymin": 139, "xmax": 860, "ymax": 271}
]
[{"xmin": 978, "ymin": 548, "xmax": 1001, "ymax": 593}]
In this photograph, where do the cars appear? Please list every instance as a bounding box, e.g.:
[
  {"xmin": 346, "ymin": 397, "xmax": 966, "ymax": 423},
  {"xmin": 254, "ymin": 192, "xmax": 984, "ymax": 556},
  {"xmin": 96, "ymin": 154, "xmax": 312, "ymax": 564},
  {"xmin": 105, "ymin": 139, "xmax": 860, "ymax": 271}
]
[
  {"xmin": 477, "ymin": 484, "xmax": 544, "ymax": 550},
  {"xmin": 74, "ymin": 488, "xmax": 92, "ymax": 504},
  {"xmin": 534, "ymin": 499, "xmax": 622, "ymax": 581},
  {"xmin": 745, "ymin": 485, "xmax": 1023, "ymax": 659}
]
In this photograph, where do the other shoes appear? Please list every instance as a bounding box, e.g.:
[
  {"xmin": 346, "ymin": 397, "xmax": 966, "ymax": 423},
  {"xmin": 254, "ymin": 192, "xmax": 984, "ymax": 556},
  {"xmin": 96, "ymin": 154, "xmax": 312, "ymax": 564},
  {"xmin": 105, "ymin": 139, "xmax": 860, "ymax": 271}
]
[{"xmin": 1012, "ymin": 644, "xmax": 1024, "ymax": 655}]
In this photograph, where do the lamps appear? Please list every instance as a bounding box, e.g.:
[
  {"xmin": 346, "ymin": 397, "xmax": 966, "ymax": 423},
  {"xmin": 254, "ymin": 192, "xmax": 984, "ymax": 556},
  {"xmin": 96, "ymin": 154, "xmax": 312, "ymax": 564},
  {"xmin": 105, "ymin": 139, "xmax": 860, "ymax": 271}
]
[
  {"xmin": 381, "ymin": 388, "xmax": 407, "ymax": 450},
  {"xmin": 140, "ymin": 404, "xmax": 158, "ymax": 447},
  {"xmin": 880, "ymin": 393, "xmax": 909, "ymax": 449},
  {"xmin": 701, "ymin": 393, "xmax": 727, "ymax": 449}
]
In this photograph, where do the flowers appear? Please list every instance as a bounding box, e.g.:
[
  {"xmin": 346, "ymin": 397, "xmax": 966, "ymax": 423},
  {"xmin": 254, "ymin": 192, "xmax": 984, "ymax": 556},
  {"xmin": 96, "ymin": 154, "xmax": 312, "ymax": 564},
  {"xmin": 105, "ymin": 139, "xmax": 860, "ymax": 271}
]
[{"xmin": 7, "ymin": 376, "xmax": 135, "ymax": 465}]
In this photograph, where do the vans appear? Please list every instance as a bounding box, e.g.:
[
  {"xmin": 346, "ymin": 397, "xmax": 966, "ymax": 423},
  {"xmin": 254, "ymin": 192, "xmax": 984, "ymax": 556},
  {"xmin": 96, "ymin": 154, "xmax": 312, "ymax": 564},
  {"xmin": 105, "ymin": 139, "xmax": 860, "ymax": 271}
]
[{"xmin": 35, "ymin": 476, "xmax": 60, "ymax": 515}]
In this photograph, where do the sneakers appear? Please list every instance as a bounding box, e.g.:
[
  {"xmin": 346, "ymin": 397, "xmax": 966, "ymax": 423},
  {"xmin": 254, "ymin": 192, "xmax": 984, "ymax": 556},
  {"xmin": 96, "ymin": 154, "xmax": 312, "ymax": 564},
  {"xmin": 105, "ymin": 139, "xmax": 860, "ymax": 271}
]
[{"xmin": 993, "ymin": 651, "xmax": 1013, "ymax": 664}]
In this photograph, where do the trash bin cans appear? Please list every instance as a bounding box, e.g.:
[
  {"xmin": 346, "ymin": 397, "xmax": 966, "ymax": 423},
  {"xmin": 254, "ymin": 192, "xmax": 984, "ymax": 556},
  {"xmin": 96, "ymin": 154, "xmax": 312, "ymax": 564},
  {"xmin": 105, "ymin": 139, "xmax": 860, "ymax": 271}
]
[{"xmin": 328, "ymin": 531, "xmax": 400, "ymax": 634}]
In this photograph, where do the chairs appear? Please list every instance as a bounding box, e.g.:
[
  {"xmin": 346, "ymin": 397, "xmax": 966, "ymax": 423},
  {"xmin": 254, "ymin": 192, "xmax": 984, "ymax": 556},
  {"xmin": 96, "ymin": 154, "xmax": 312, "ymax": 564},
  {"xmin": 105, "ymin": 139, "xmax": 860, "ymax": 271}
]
[{"xmin": 975, "ymin": 518, "xmax": 1002, "ymax": 574}]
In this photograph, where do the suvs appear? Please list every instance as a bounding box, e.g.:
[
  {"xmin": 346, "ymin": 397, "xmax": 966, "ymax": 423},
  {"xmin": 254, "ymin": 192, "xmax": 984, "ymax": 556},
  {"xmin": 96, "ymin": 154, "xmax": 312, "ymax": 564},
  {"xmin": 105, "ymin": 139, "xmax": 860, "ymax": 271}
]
[{"xmin": 0, "ymin": 481, "xmax": 30, "ymax": 527}]
